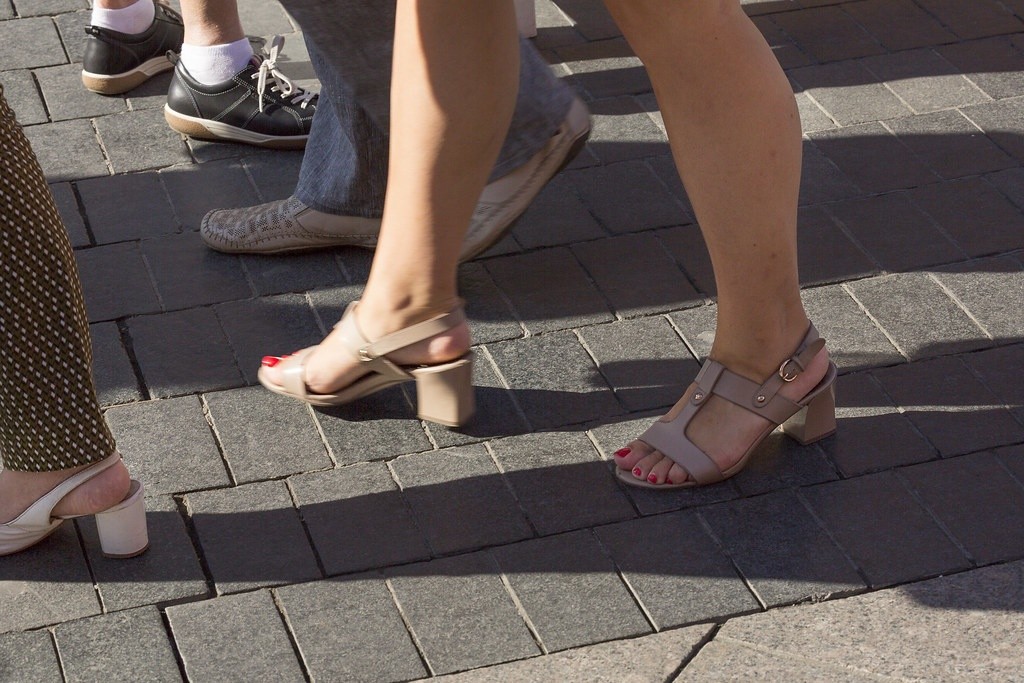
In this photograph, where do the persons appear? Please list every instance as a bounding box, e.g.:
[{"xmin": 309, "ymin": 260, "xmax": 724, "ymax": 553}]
[
  {"xmin": 83, "ymin": 0, "xmax": 836, "ymax": 484},
  {"xmin": 0, "ymin": 85, "xmax": 151, "ymax": 560}
]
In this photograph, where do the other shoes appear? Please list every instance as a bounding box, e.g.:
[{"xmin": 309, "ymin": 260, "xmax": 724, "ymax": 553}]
[
  {"xmin": 201, "ymin": 192, "xmax": 379, "ymax": 255},
  {"xmin": 456, "ymin": 96, "xmax": 594, "ymax": 262}
]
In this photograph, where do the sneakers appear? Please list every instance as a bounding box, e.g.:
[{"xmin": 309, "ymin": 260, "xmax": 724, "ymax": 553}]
[
  {"xmin": 81, "ymin": 2, "xmax": 186, "ymax": 94},
  {"xmin": 165, "ymin": 35, "xmax": 322, "ymax": 146}
]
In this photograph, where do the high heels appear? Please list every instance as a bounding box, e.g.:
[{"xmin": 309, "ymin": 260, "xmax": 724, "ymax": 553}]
[
  {"xmin": 617, "ymin": 321, "xmax": 842, "ymax": 490},
  {"xmin": 258, "ymin": 300, "xmax": 477, "ymax": 429},
  {"xmin": 1, "ymin": 448, "xmax": 151, "ymax": 560}
]
